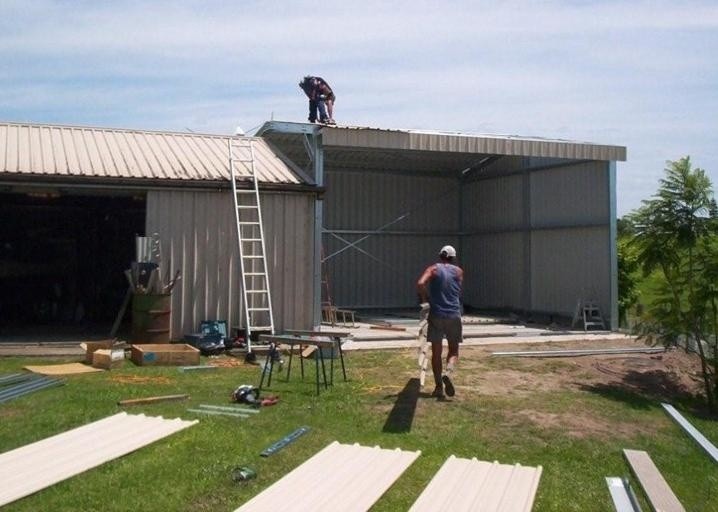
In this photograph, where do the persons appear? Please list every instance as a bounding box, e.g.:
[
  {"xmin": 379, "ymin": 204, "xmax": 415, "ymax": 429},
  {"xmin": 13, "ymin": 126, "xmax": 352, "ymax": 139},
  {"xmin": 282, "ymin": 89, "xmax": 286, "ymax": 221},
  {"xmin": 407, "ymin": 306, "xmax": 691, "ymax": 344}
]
[
  {"xmin": 419, "ymin": 244, "xmax": 465, "ymax": 397},
  {"xmin": 305, "ymin": 75, "xmax": 334, "ymax": 123}
]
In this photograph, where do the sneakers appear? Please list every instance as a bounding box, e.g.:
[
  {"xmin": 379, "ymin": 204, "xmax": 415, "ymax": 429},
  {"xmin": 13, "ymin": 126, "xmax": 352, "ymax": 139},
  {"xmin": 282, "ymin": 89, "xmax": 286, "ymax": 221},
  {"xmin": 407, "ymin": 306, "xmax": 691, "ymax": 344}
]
[
  {"xmin": 442, "ymin": 374, "xmax": 455, "ymax": 396},
  {"xmin": 433, "ymin": 384, "xmax": 442, "ymax": 397}
]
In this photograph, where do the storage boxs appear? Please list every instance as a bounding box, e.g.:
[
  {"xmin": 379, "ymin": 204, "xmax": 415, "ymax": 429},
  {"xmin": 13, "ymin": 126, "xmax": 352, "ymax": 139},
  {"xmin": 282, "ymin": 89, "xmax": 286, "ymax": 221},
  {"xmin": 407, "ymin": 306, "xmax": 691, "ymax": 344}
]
[{"xmin": 79, "ymin": 339, "xmax": 127, "ymax": 370}]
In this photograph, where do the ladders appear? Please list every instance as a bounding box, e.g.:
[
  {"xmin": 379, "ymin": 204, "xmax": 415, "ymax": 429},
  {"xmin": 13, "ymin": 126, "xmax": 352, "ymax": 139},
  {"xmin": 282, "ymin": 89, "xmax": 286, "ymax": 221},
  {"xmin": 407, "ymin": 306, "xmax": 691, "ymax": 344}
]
[
  {"xmin": 322, "ymin": 231, "xmax": 357, "ymax": 327},
  {"xmin": 229, "ymin": 139, "xmax": 277, "ymax": 353}
]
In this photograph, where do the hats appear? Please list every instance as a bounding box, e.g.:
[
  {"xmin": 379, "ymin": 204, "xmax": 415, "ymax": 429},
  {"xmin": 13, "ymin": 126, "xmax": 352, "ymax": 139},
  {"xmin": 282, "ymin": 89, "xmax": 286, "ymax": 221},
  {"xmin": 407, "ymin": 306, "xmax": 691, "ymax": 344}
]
[{"xmin": 439, "ymin": 245, "xmax": 457, "ymax": 257}]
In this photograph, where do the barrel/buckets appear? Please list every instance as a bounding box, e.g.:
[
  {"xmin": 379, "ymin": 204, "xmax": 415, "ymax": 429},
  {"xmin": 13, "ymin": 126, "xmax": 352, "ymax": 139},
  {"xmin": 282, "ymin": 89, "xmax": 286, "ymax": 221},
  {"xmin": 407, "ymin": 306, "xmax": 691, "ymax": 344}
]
[{"xmin": 132, "ymin": 293, "xmax": 171, "ymax": 344}]
